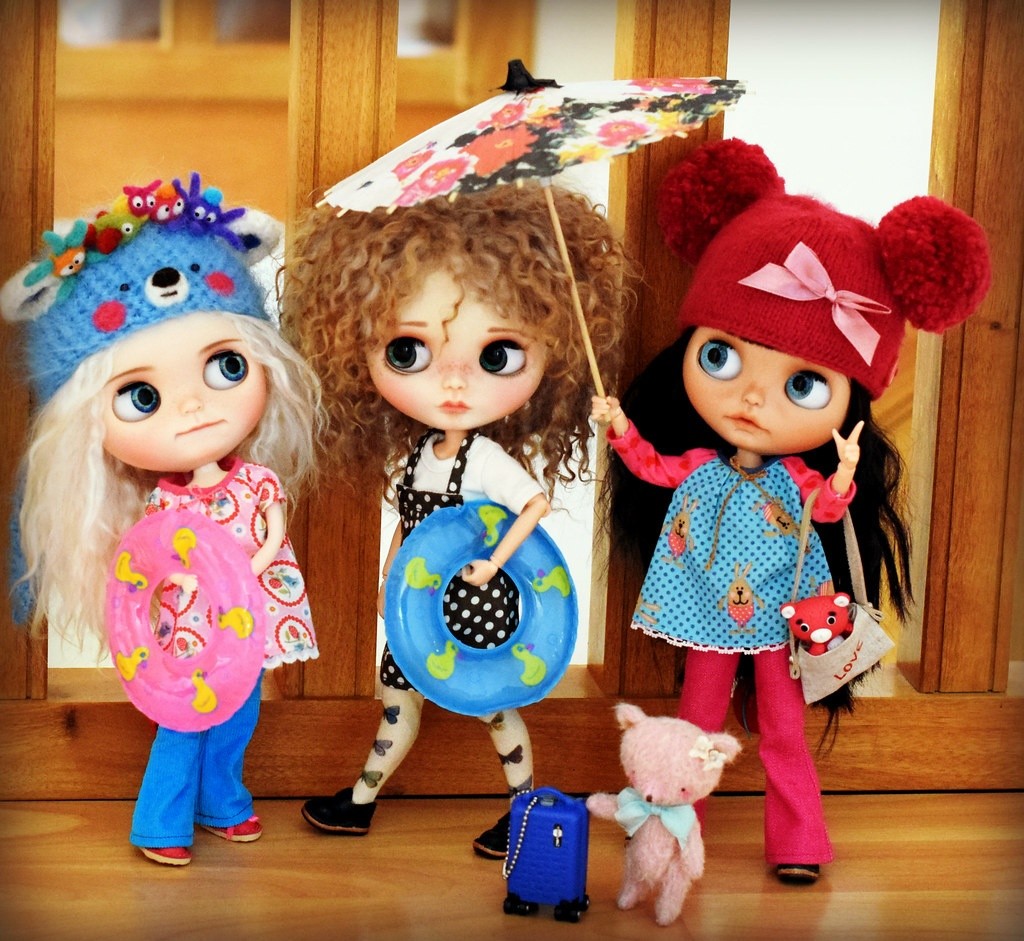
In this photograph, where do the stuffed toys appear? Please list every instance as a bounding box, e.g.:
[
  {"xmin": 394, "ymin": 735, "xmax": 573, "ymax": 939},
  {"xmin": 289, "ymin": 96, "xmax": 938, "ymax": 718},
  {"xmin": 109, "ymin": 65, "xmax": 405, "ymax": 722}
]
[{"xmin": 586, "ymin": 703, "xmax": 740, "ymax": 927}]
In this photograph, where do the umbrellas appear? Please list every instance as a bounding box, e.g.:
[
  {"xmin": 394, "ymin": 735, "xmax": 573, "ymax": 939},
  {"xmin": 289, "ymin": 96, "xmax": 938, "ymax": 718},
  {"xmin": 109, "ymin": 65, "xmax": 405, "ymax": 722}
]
[{"xmin": 314, "ymin": 58, "xmax": 749, "ymax": 422}]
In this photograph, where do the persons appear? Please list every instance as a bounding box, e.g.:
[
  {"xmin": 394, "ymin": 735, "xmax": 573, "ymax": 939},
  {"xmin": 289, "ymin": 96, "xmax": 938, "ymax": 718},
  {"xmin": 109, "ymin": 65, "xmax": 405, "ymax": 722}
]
[
  {"xmin": 590, "ymin": 140, "xmax": 988, "ymax": 885},
  {"xmin": 289, "ymin": 186, "xmax": 624, "ymax": 860},
  {"xmin": 0, "ymin": 174, "xmax": 327, "ymax": 867}
]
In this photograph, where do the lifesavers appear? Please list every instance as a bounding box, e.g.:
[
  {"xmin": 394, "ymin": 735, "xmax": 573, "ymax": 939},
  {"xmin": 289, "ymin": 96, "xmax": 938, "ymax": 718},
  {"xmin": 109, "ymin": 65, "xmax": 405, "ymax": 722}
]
[
  {"xmin": 105, "ymin": 508, "xmax": 267, "ymax": 733},
  {"xmin": 381, "ymin": 501, "xmax": 580, "ymax": 718}
]
